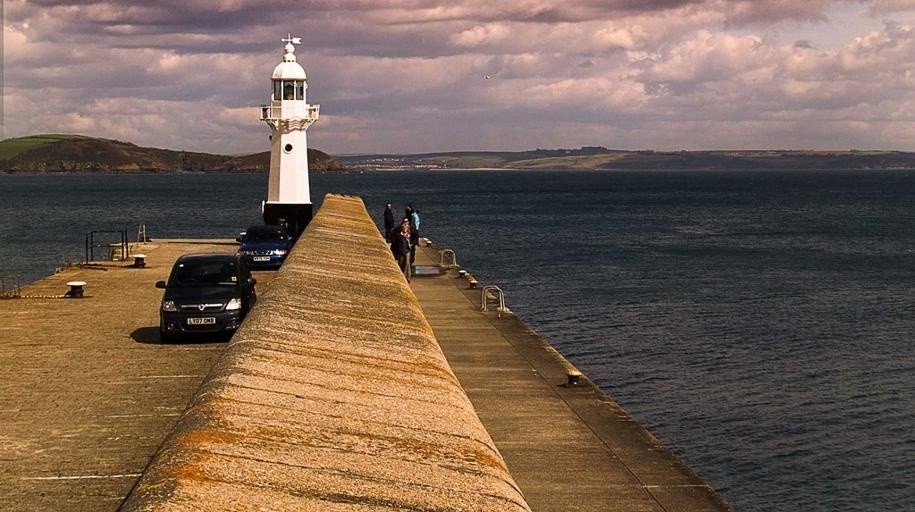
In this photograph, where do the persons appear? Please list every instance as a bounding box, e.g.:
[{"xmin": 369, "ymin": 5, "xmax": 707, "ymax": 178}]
[{"xmin": 383, "ymin": 201, "xmax": 421, "ymax": 284}]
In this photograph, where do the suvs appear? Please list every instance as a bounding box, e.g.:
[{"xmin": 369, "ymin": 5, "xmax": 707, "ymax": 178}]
[
  {"xmin": 234, "ymin": 223, "xmax": 295, "ymax": 271},
  {"xmin": 155, "ymin": 252, "xmax": 258, "ymax": 340}
]
